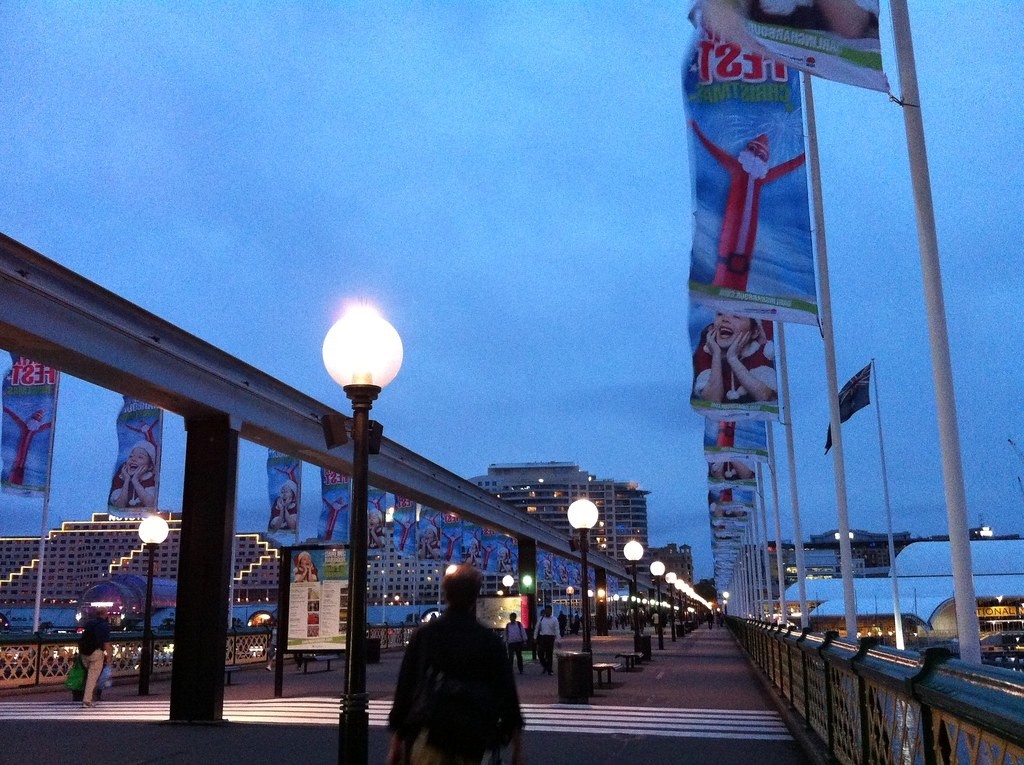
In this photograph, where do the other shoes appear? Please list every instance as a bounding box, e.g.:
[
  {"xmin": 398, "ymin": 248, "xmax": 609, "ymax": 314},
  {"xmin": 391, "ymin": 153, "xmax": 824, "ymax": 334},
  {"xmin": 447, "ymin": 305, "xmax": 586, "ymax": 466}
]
[
  {"xmin": 548, "ymin": 672, "xmax": 554, "ymax": 675},
  {"xmin": 82, "ymin": 701, "xmax": 96, "ymax": 708}
]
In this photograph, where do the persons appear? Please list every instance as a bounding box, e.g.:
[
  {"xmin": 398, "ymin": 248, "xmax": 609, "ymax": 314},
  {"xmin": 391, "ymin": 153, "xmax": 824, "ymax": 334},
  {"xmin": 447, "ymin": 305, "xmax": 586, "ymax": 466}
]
[
  {"xmin": 294, "ymin": 551, "xmax": 318, "ymax": 582},
  {"xmin": 498, "ymin": 548, "xmax": 512, "ymax": 572},
  {"xmin": 534, "ymin": 605, "xmax": 726, "ymax": 675},
  {"xmin": 503, "ymin": 613, "xmax": 529, "ymax": 674},
  {"xmin": 711, "ymin": 462, "xmax": 755, "ymax": 480},
  {"xmin": 265, "ymin": 619, "xmax": 303, "ymax": 671},
  {"xmin": 268, "ymin": 481, "xmax": 296, "ymax": 531},
  {"xmin": 110, "ymin": 439, "xmax": 158, "ymax": 507},
  {"xmin": 465, "ymin": 538, "xmax": 480, "ymax": 565},
  {"xmin": 386, "ymin": 565, "xmax": 524, "ymax": 765},
  {"xmin": 418, "ymin": 525, "xmax": 441, "ymax": 559},
  {"xmin": 695, "ymin": 310, "xmax": 780, "ymax": 401},
  {"xmin": 79, "ymin": 607, "xmax": 112, "ymax": 708},
  {"xmin": 368, "ymin": 508, "xmax": 385, "ymax": 548}
]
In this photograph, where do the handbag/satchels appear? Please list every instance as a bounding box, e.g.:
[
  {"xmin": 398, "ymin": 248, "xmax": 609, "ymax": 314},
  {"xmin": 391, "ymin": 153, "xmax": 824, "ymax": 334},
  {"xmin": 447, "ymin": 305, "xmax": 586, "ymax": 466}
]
[
  {"xmin": 537, "ymin": 635, "xmax": 543, "ymax": 651},
  {"xmin": 65, "ymin": 655, "xmax": 88, "ymax": 690},
  {"xmin": 98, "ymin": 664, "xmax": 113, "ymax": 689}
]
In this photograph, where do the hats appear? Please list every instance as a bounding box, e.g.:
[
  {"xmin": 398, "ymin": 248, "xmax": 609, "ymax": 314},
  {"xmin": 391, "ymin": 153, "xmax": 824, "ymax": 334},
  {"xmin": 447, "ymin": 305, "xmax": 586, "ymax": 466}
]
[
  {"xmin": 282, "ymin": 479, "xmax": 298, "ymax": 498},
  {"xmin": 755, "ymin": 316, "xmax": 773, "ymax": 344},
  {"xmin": 370, "ymin": 507, "xmax": 382, "ymax": 522},
  {"xmin": 293, "ymin": 550, "xmax": 311, "ymax": 573},
  {"xmin": 471, "ymin": 537, "xmax": 479, "ymax": 548},
  {"xmin": 498, "ymin": 547, "xmax": 579, "ymax": 575},
  {"xmin": 424, "ymin": 524, "xmax": 438, "ymax": 537},
  {"xmin": 130, "ymin": 439, "xmax": 157, "ymax": 466}
]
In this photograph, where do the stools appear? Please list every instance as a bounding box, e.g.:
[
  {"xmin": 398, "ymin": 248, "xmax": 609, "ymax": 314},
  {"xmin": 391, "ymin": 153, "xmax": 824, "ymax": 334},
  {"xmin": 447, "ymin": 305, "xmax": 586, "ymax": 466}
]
[
  {"xmin": 616, "ymin": 653, "xmax": 646, "ymax": 671},
  {"xmin": 593, "ymin": 663, "xmax": 623, "ymax": 687}
]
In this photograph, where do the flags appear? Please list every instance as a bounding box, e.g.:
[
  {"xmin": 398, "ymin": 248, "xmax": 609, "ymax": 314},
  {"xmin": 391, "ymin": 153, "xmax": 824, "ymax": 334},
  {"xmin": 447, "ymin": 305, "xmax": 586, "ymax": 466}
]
[{"xmin": 825, "ymin": 362, "xmax": 871, "ymax": 454}]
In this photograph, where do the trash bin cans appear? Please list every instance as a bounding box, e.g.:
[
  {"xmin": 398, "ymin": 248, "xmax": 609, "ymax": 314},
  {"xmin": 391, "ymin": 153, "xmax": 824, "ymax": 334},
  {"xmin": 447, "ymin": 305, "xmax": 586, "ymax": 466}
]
[
  {"xmin": 367, "ymin": 638, "xmax": 381, "ymax": 663},
  {"xmin": 633, "ymin": 634, "xmax": 652, "ymax": 661},
  {"xmin": 676, "ymin": 624, "xmax": 684, "ymax": 637},
  {"xmin": 73, "ymin": 656, "xmax": 99, "ymax": 701},
  {"xmin": 554, "ymin": 652, "xmax": 593, "ymax": 704}
]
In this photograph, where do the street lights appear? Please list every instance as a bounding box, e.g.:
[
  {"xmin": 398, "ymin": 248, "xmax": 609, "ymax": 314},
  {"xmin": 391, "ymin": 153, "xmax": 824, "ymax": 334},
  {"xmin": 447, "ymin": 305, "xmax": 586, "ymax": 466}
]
[
  {"xmin": 567, "ymin": 586, "xmax": 574, "ymax": 634},
  {"xmin": 588, "ymin": 590, "xmax": 593, "ymax": 632},
  {"xmin": 624, "ymin": 540, "xmax": 644, "ymax": 663},
  {"xmin": 665, "ymin": 572, "xmax": 676, "ymax": 642},
  {"xmin": 136, "ymin": 517, "xmax": 170, "ymax": 695},
  {"xmin": 503, "ymin": 575, "xmax": 514, "ymax": 596},
  {"xmin": 674, "ymin": 579, "xmax": 712, "ymax": 634},
  {"xmin": 321, "ymin": 303, "xmax": 404, "ymax": 765},
  {"xmin": 568, "ymin": 499, "xmax": 598, "ymax": 696},
  {"xmin": 650, "ymin": 561, "xmax": 666, "ymax": 650},
  {"xmin": 614, "ymin": 594, "xmax": 619, "ymax": 630}
]
